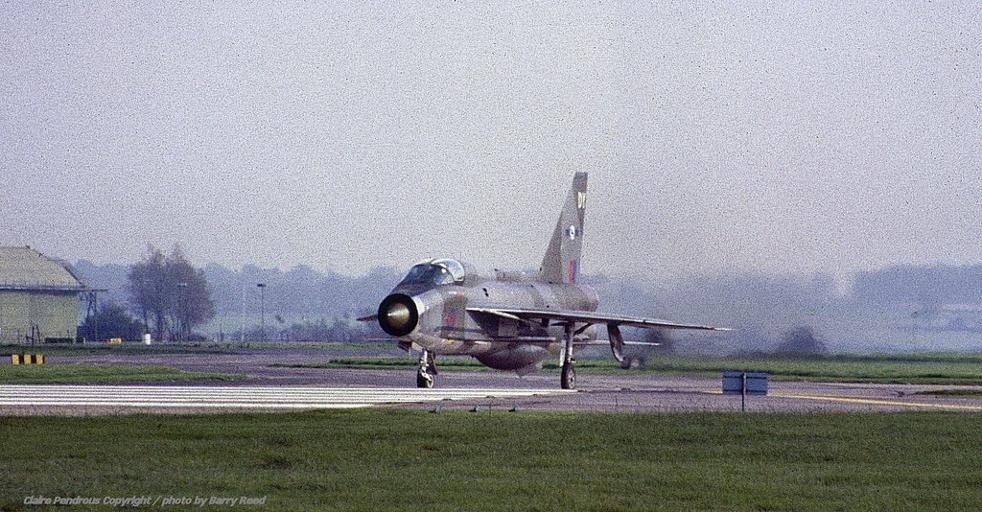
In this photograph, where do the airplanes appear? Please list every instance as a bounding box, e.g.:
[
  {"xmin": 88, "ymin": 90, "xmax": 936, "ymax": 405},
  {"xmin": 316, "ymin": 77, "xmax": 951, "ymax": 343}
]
[{"xmin": 354, "ymin": 169, "xmax": 745, "ymax": 390}]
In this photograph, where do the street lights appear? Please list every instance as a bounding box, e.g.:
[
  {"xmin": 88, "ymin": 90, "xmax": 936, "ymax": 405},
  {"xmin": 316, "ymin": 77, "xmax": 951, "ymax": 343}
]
[
  {"xmin": 257, "ymin": 282, "xmax": 268, "ymax": 342},
  {"xmin": 176, "ymin": 283, "xmax": 189, "ymax": 340}
]
